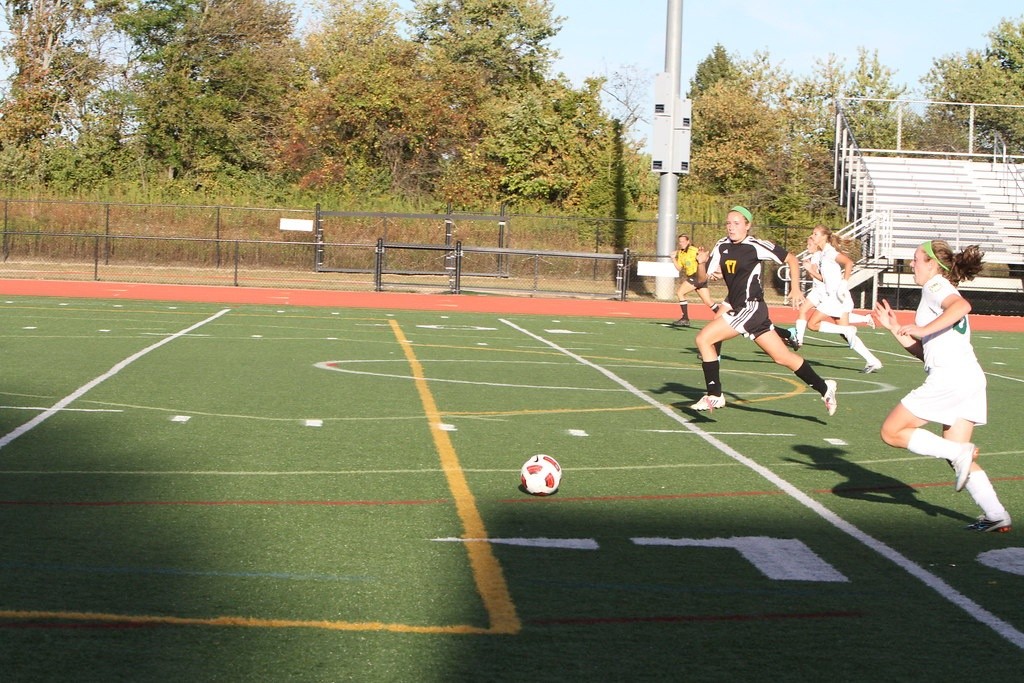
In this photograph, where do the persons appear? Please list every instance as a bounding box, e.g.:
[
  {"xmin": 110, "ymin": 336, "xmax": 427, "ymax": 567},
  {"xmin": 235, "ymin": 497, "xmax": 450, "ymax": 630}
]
[
  {"xmin": 688, "ymin": 205, "xmax": 837, "ymax": 417},
  {"xmin": 802, "ymin": 224, "xmax": 883, "ymax": 374},
  {"xmin": 873, "ymin": 240, "xmax": 1013, "ymax": 534},
  {"xmin": 669, "ymin": 233, "xmax": 720, "ymax": 327},
  {"xmin": 698, "ymin": 324, "xmax": 799, "ymax": 362},
  {"xmin": 789, "ymin": 236, "xmax": 877, "ymax": 347}
]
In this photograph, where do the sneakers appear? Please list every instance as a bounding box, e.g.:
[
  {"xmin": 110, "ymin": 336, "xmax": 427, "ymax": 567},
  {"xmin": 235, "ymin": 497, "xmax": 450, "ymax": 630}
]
[
  {"xmin": 782, "ymin": 338, "xmax": 802, "ymax": 348},
  {"xmin": 787, "ymin": 327, "xmax": 799, "ymax": 351},
  {"xmin": 951, "ymin": 442, "xmax": 979, "ymax": 492},
  {"xmin": 821, "ymin": 379, "xmax": 838, "ymax": 417},
  {"xmin": 862, "ymin": 359, "xmax": 884, "ymax": 375},
  {"xmin": 673, "ymin": 318, "xmax": 690, "ymax": 327},
  {"xmin": 697, "ymin": 354, "xmax": 721, "ymax": 363},
  {"xmin": 690, "ymin": 393, "xmax": 725, "ymax": 411},
  {"xmin": 962, "ymin": 511, "xmax": 1012, "ymax": 533},
  {"xmin": 844, "ymin": 326, "xmax": 857, "ymax": 350},
  {"xmin": 866, "ymin": 314, "xmax": 875, "ymax": 329}
]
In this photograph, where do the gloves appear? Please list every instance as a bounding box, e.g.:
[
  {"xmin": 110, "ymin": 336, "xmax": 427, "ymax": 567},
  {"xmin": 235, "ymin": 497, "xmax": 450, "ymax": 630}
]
[{"xmin": 837, "ymin": 279, "xmax": 848, "ymax": 303}]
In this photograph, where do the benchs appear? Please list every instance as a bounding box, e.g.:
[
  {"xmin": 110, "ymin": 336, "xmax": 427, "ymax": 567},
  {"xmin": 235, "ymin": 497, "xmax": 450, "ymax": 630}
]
[{"xmin": 842, "ymin": 154, "xmax": 1024, "ymax": 264}]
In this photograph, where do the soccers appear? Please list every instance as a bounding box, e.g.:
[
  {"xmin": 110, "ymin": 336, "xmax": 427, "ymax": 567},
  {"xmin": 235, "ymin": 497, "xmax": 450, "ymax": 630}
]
[{"xmin": 520, "ymin": 453, "xmax": 562, "ymax": 497}]
